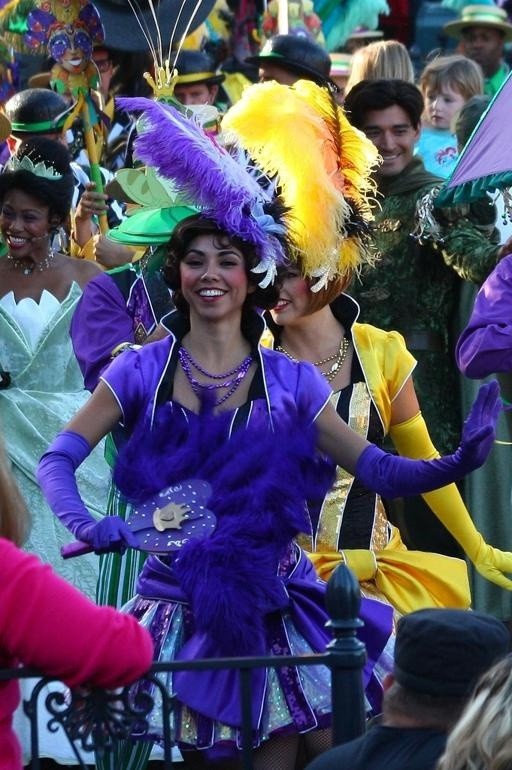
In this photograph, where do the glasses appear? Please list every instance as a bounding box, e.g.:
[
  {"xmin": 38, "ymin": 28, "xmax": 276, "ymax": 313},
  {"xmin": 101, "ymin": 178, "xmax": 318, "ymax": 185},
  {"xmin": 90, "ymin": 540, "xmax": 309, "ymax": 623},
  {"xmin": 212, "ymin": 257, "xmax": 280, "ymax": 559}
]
[{"xmin": 96, "ymin": 57, "xmax": 114, "ymax": 74}]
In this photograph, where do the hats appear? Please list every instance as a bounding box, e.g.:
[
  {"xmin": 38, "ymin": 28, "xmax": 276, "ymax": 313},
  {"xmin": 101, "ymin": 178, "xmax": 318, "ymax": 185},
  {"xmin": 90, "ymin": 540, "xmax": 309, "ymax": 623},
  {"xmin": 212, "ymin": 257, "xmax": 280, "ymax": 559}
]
[
  {"xmin": 440, "ymin": 2, "xmax": 512, "ymax": 44},
  {"xmin": 243, "ymin": 32, "xmax": 341, "ymax": 93},
  {"xmin": 0, "ymin": 87, "xmax": 78, "ymax": 138},
  {"xmin": 391, "ymin": 606, "xmax": 512, "ymax": 701},
  {"xmin": 156, "ymin": 48, "xmax": 229, "ymax": 89}
]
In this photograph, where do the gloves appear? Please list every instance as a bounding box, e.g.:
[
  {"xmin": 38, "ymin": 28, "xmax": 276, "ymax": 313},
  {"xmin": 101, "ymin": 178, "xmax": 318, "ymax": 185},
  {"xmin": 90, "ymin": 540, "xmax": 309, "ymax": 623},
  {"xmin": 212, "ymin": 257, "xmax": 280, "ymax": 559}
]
[
  {"xmin": 384, "ymin": 410, "xmax": 512, "ymax": 591},
  {"xmin": 32, "ymin": 430, "xmax": 142, "ymax": 556},
  {"xmin": 353, "ymin": 376, "xmax": 505, "ymax": 500}
]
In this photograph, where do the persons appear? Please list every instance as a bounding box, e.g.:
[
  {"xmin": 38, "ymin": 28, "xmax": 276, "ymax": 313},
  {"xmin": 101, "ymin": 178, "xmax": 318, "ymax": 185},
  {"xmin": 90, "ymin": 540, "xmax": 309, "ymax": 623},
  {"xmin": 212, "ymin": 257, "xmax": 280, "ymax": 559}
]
[{"xmin": 0, "ymin": 0, "xmax": 512, "ymax": 769}]
